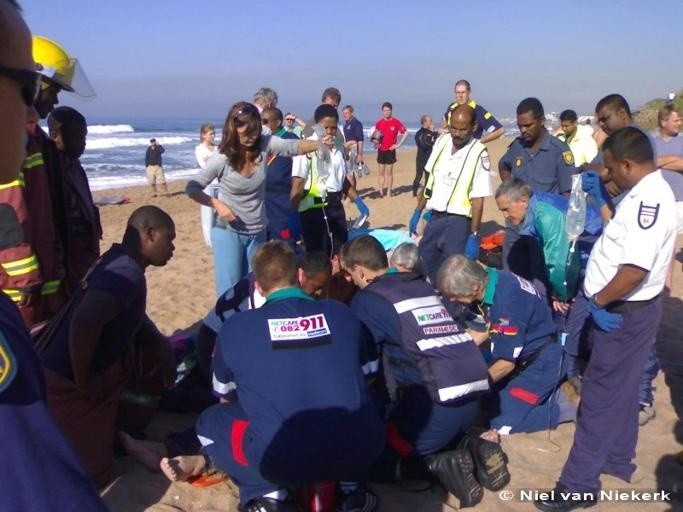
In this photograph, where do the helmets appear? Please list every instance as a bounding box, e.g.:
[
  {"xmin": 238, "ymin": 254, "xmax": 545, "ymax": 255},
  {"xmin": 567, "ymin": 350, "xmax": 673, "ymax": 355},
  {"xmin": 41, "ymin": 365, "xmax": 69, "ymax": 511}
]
[{"xmin": 32, "ymin": 36, "xmax": 75, "ymax": 91}]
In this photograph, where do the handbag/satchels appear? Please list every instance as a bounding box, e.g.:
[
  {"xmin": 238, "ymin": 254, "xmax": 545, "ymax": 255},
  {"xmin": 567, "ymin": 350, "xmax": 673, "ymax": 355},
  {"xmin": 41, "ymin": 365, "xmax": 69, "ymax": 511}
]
[{"xmin": 32, "ymin": 342, "xmax": 125, "ymax": 487}]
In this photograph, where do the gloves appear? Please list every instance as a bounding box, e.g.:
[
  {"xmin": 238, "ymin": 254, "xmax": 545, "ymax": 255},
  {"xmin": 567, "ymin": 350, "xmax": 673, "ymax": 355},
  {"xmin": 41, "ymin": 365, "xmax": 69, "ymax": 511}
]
[
  {"xmin": 464, "ymin": 235, "xmax": 480, "ymax": 259},
  {"xmin": 409, "ymin": 208, "xmax": 422, "ymax": 236},
  {"xmin": 353, "ymin": 196, "xmax": 369, "ymax": 217},
  {"xmin": 587, "ymin": 298, "xmax": 624, "ymax": 332}
]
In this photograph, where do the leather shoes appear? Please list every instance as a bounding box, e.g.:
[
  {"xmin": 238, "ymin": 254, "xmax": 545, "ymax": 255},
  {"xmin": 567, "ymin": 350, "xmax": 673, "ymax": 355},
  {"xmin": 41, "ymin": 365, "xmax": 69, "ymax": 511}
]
[{"xmin": 534, "ymin": 481, "xmax": 597, "ymax": 512}]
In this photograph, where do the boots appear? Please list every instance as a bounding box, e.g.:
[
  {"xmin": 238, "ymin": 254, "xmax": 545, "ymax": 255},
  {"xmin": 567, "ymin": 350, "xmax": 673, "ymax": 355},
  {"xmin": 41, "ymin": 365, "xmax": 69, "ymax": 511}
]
[{"xmin": 427, "ymin": 436, "xmax": 510, "ymax": 508}]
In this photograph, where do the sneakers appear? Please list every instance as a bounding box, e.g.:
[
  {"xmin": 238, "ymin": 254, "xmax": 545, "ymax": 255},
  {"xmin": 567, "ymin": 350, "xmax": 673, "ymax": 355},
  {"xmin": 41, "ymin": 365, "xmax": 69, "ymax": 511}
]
[
  {"xmin": 355, "ymin": 162, "xmax": 369, "ymax": 176},
  {"xmin": 637, "ymin": 402, "xmax": 655, "ymax": 425},
  {"xmin": 335, "ymin": 483, "xmax": 379, "ymax": 512}
]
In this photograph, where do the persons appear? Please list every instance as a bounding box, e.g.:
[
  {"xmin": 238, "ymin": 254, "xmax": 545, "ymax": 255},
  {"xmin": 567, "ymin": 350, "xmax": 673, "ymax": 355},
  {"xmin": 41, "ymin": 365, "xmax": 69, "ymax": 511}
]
[{"xmin": 0, "ymin": 0, "xmax": 683, "ymax": 512}]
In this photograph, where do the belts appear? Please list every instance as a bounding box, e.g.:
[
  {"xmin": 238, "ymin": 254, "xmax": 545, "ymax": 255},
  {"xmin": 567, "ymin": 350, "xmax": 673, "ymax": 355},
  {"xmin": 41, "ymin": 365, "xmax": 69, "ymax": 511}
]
[{"xmin": 611, "ymin": 300, "xmax": 652, "ymax": 314}]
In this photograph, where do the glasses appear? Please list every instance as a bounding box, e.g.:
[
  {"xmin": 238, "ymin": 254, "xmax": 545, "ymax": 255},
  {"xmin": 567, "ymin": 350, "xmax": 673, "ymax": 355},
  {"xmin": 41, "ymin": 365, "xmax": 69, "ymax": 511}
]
[
  {"xmin": 1, "ymin": 64, "xmax": 42, "ymax": 107},
  {"xmin": 235, "ymin": 104, "xmax": 257, "ymax": 119}
]
